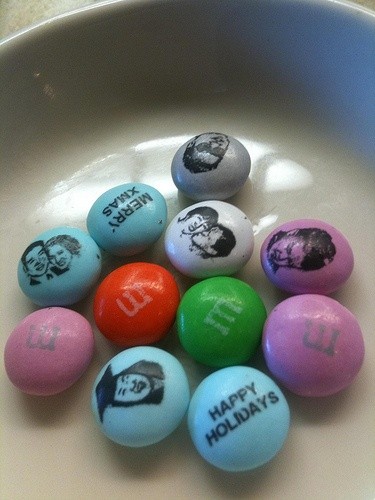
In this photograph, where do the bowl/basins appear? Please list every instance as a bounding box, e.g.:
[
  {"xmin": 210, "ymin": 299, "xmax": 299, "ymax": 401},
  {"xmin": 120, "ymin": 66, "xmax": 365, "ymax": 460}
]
[{"xmin": 0, "ymin": 0, "xmax": 375, "ymax": 500}]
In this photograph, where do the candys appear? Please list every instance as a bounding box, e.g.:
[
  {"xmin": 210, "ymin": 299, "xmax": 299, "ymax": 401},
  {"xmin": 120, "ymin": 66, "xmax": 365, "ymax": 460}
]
[{"xmin": 4, "ymin": 130, "xmax": 367, "ymax": 473}]
